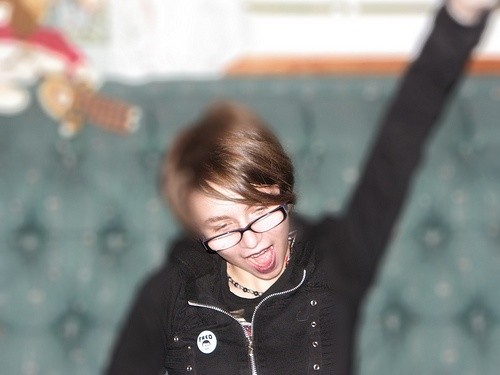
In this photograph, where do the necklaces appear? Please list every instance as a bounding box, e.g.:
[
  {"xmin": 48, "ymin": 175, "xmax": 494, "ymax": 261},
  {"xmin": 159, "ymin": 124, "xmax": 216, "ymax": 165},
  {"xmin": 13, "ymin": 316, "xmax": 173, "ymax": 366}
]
[{"xmin": 227, "ymin": 236, "xmax": 293, "ymax": 298}]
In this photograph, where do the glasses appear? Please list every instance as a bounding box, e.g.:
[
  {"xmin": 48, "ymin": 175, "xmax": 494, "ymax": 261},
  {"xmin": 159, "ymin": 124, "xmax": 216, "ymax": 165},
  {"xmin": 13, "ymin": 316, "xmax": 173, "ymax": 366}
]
[{"xmin": 197, "ymin": 200, "xmax": 291, "ymax": 254}]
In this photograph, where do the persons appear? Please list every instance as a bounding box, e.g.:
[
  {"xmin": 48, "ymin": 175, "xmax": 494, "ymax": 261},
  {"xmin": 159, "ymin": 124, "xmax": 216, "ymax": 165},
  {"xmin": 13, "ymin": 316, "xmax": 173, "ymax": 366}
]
[{"xmin": 101, "ymin": 0, "xmax": 500, "ymax": 375}]
